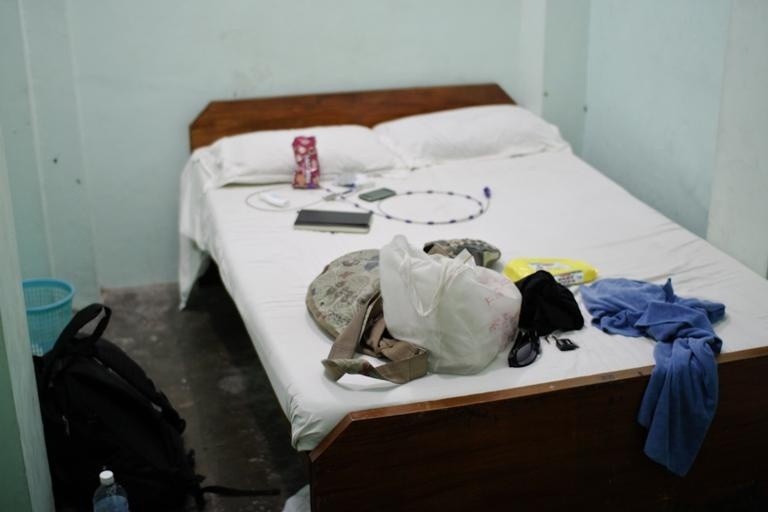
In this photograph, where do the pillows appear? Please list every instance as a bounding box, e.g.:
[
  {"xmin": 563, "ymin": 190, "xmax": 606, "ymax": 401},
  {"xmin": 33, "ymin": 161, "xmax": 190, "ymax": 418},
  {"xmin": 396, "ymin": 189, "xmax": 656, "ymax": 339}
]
[
  {"xmin": 372, "ymin": 104, "xmax": 566, "ymax": 173},
  {"xmin": 197, "ymin": 123, "xmax": 406, "ymax": 188}
]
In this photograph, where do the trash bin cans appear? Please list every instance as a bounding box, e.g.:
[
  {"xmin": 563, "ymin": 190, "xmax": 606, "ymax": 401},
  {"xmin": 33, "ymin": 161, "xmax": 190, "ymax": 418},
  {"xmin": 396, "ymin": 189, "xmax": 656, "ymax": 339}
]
[{"xmin": 22, "ymin": 279, "xmax": 76, "ymax": 356}]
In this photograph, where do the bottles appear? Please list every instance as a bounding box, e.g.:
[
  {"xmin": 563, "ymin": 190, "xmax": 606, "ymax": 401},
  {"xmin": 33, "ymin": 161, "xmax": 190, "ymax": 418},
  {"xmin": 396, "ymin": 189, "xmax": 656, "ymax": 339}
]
[{"xmin": 92, "ymin": 471, "xmax": 128, "ymax": 512}]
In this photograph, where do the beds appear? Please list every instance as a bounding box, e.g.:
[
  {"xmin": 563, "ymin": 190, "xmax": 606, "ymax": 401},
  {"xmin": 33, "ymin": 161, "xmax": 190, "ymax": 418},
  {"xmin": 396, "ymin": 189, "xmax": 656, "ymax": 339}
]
[{"xmin": 187, "ymin": 82, "xmax": 767, "ymax": 512}]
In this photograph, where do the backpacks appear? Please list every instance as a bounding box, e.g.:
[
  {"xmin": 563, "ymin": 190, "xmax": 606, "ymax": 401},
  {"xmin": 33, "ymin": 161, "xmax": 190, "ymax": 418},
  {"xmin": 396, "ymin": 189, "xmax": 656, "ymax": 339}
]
[{"xmin": 31, "ymin": 303, "xmax": 281, "ymax": 512}]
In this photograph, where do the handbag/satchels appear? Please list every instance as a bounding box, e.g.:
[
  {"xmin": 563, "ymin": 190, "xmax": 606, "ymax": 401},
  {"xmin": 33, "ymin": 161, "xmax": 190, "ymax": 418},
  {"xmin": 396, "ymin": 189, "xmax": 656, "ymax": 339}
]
[
  {"xmin": 377, "ymin": 236, "xmax": 521, "ymax": 375},
  {"xmin": 306, "ymin": 238, "xmax": 501, "ymax": 385},
  {"xmin": 508, "ymin": 270, "xmax": 584, "ymax": 368}
]
